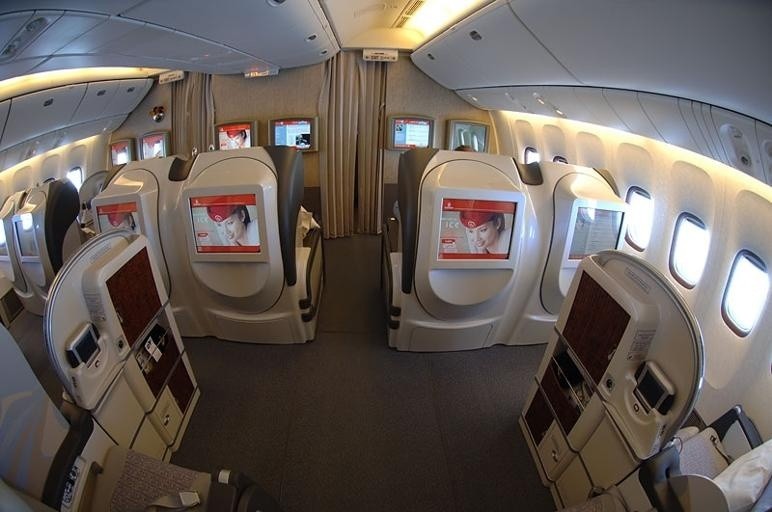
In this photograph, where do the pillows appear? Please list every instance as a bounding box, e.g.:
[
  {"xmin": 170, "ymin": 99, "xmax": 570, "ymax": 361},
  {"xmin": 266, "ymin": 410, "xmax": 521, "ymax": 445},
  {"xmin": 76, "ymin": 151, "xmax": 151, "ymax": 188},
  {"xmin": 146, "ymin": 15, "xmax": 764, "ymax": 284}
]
[{"xmin": 709, "ymin": 438, "xmax": 771, "ymax": 512}]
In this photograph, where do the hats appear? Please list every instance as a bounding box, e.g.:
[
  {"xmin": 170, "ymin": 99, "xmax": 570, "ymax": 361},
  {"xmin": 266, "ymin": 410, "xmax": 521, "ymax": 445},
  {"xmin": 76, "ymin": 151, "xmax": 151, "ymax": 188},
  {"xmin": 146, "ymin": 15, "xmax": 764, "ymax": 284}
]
[
  {"xmin": 226, "ymin": 130, "xmax": 242, "ymax": 138},
  {"xmin": 460, "ymin": 211, "xmax": 495, "ymax": 228},
  {"xmin": 207, "ymin": 205, "xmax": 236, "ymax": 222},
  {"xmin": 108, "ymin": 214, "xmax": 126, "ymax": 228}
]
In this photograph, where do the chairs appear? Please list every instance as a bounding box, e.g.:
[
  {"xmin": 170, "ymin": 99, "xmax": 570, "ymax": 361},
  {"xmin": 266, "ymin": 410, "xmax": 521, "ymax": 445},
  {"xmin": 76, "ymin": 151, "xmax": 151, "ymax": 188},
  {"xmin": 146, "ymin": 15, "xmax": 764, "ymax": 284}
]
[
  {"xmin": 378, "ymin": 147, "xmax": 630, "ymax": 349},
  {"xmin": 620, "ymin": 404, "xmax": 771, "ymax": 512},
  {"xmin": 3, "ymin": 178, "xmax": 80, "ymax": 318},
  {"xmin": 92, "ymin": 146, "xmax": 324, "ymax": 344},
  {"xmin": 70, "ymin": 442, "xmax": 281, "ymax": 511}
]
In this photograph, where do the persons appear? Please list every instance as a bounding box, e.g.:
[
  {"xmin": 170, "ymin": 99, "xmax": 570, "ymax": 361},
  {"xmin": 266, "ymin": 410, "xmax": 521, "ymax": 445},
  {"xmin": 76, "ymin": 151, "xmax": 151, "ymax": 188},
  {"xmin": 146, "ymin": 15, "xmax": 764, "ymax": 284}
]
[
  {"xmin": 226, "ymin": 130, "xmax": 250, "ymax": 149},
  {"xmin": 206, "ymin": 205, "xmax": 259, "ymax": 246},
  {"xmin": 459, "ymin": 210, "xmax": 510, "ymax": 254},
  {"xmin": 107, "ymin": 213, "xmax": 140, "ymax": 234}
]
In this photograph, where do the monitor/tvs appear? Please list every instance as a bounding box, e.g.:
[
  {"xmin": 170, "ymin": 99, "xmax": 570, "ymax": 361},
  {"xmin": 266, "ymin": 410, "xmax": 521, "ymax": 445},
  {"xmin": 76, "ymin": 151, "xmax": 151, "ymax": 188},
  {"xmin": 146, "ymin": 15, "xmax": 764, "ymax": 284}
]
[
  {"xmin": 446, "ymin": 120, "xmax": 491, "ymax": 154},
  {"xmin": 104, "ymin": 245, "xmax": 165, "ymax": 351},
  {"xmin": 12, "ymin": 210, "xmax": 39, "ymax": 263},
  {"xmin": 385, "ymin": 115, "xmax": 435, "ymax": 152},
  {"xmin": 180, "ymin": 184, "xmax": 267, "ymax": 265},
  {"xmin": 561, "ymin": 201, "xmax": 632, "ymax": 267},
  {"xmin": 137, "ymin": 130, "xmax": 171, "ymax": 160},
  {"xmin": 0, "ymin": 217, "xmax": 9, "ymax": 260},
  {"xmin": 267, "ymin": 115, "xmax": 319, "ymax": 153},
  {"xmin": 108, "ymin": 137, "xmax": 136, "ymax": 168},
  {"xmin": 430, "ymin": 187, "xmax": 528, "ymax": 270},
  {"xmin": 90, "ymin": 193, "xmax": 142, "ymax": 232},
  {"xmin": 561, "ymin": 270, "xmax": 632, "ymax": 386},
  {"xmin": 215, "ymin": 119, "xmax": 258, "ymax": 151}
]
